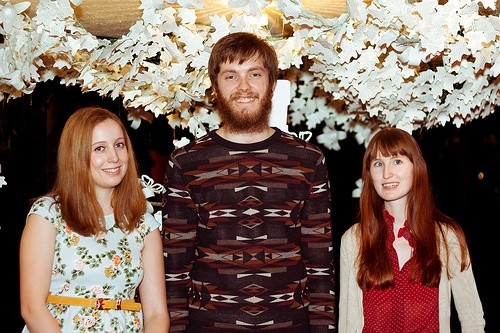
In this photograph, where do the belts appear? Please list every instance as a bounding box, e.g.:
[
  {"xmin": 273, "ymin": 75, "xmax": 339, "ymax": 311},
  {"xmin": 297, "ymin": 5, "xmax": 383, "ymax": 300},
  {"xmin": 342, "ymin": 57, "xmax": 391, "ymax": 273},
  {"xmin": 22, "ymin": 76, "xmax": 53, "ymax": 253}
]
[{"xmin": 46, "ymin": 295, "xmax": 141, "ymax": 311}]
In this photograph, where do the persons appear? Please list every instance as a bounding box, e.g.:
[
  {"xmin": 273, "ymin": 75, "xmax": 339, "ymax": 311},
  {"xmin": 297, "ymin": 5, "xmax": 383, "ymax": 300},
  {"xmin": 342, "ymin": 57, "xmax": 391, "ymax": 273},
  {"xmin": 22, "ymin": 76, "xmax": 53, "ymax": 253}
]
[
  {"xmin": 337, "ymin": 128, "xmax": 486, "ymax": 333},
  {"xmin": 18, "ymin": 106, "xmax": 170, "ymax": 332},
  {"xmin": 163, "ymin": 33, "xmax": 336, "ymax": 333}
]
